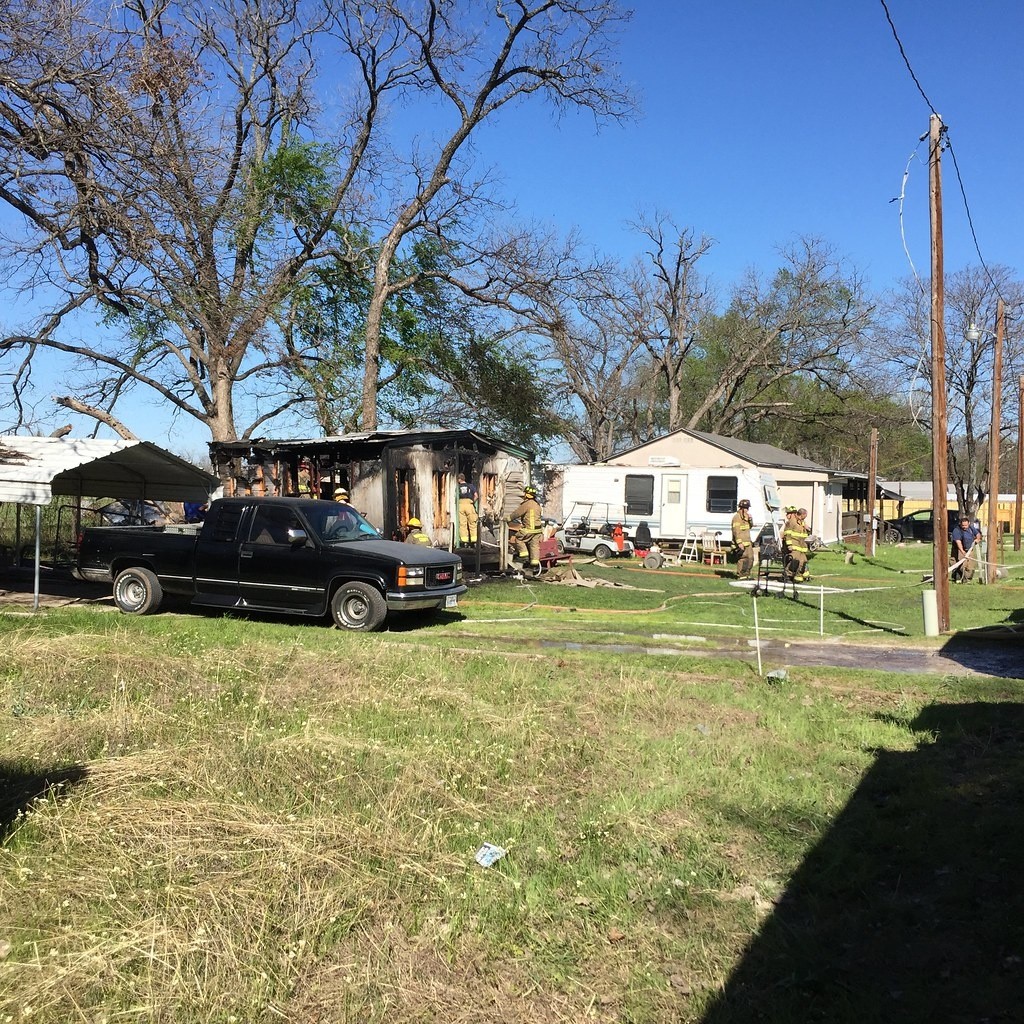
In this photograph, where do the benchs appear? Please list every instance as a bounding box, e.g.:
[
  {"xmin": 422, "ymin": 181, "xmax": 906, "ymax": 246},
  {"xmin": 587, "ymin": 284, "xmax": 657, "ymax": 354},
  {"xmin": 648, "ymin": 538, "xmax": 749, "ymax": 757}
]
[{"xmin": 528, "ymin": 541, "xmax": 574, "ymax": 572}]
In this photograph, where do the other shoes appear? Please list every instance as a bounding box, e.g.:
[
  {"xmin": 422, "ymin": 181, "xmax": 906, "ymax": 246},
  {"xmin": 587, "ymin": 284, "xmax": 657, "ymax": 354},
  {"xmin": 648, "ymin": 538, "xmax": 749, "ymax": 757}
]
[
  {"xmin": 737, "ymin": 574, "xmax": 754, "ymax": 581},
  {"xmin": 804, "ymin": 576, "xmax": 812, "ymax": 582},
  {"xmin": 526, "ymin": 565, "xmax": 539, "ymax": 571},
  {"xmin": 471, "ymin": 542, "xmax": 476, "ymax": 548},
  {"xmin": 463, "ymin": 542, "xmax": 468, "ymax": 547},
  {"xmin": 782, "ymin": 572, "xmax": 791, "ymax": 580},
  {"xmin": 514, "ymin": 556, "xmax": 529, "ymax": 562}
]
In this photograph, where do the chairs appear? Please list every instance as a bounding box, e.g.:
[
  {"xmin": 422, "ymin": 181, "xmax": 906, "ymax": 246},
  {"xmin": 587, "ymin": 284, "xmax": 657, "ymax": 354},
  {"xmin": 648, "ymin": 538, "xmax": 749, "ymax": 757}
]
[
  {"xmin": 250, "ymin": 510, "xmax": 295, "ymax": 544},
  {"xmin": 635, "ymin": 521, "xmax": 659, "ymax": 548},
  {"xmin": 599, "ymin": 525, "xmax": 609, "ymax": 533},
  {"xmin": 701, "ymin": 531, "xmax": 726, "ymax": 566}
]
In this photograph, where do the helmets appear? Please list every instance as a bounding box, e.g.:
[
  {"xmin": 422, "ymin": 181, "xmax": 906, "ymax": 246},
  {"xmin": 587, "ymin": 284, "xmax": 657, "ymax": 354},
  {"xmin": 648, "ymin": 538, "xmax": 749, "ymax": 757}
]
[
  {"xmin": 739, "ymin": 499, "xmax": 751, "ymax": 508},
  {"xmin": 785, "ymin": 506, "xmax": 798, "ymax": 513},
  {"xmin": 406, "ymin": 518, "xmax": 422, "ymax": 528},
  {"xmin": 523, "ymin": 486, "xmax": 537, "ymax": 497},
  {"xmin": 332, "ymin": 488, "xmax": 349, "ymax": 500}
]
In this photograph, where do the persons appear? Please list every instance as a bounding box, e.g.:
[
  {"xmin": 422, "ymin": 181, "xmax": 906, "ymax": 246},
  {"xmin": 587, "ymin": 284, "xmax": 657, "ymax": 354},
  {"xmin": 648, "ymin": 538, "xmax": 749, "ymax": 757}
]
[
  {"xmin": 457, "ymin": 473, "xmax": 478, "ymax": 548},
  {"xmin": 325, "ymin": 488, "xmax": 357, "ymax": 535},
  {"xmin": 780, "ymin": 506, "xmax": 811, "ymax": 584},
  {"xmin": 184, "ymin": 502, "xmax": 208, "ymax": 523},
  {"xmin": 404, "ymin": 517, "xmax": 432, "ymax": 548},
  {"xmin": 730, "ymin": 499, "xmax": 754, "ymax": 579},
  {"xmin": 949, "ymin": 518, "xmax": 980, "ymax": 583},
  {"xmin": 502, "ymin": 486, "xmax": 542, "ymax": 575}
]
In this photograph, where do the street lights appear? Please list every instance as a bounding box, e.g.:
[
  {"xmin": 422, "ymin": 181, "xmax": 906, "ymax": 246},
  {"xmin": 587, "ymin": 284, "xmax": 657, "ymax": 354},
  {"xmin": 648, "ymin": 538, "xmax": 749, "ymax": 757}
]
[{"xmin": 965, "ymin": 297, "xmax": 1005, "ymax": 584}]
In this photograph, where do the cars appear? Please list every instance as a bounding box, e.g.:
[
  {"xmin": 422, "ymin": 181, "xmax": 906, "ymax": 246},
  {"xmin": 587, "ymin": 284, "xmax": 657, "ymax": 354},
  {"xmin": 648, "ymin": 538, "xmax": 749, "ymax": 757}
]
[
  {"xmin": 876, "ymin": 508, "xmax": 981, "ymax": 544},
  {"xmin": 549, "ymin": 516, "xmax": 634, "ymax": 562}
]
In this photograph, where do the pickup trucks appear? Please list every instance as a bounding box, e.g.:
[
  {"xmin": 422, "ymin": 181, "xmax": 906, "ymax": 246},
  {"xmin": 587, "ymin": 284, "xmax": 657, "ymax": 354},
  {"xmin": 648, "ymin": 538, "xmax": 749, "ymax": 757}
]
[{"xmin": 68, "ymin": 497, "xmax": 468, "ymax": 633}]
